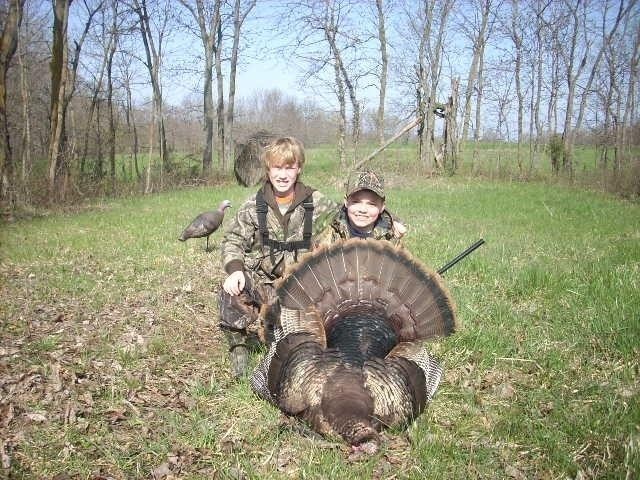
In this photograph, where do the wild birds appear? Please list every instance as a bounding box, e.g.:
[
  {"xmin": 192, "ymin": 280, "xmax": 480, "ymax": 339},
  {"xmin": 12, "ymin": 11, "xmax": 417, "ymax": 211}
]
[{"xmin": 178, "ymin": 200, "xmax": 233, "ymax": 252}]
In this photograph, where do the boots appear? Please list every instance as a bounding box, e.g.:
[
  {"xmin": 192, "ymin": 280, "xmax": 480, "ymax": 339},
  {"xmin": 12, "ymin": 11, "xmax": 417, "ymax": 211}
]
[{"xmin": 222, "ymin": 327, "xmax": 248, "ymax": 376}]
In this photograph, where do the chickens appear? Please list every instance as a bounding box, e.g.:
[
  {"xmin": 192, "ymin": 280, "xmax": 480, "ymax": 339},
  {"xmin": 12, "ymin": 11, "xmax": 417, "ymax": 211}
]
[{"xmin": 248, "ymin": 236, "xmax": 458, "ymax": 469}]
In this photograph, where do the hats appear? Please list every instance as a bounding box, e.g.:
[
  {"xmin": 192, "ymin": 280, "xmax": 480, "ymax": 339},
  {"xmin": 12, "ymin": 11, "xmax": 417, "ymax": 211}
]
[{"xmin": 346, "ymin": 168, "xmax": 386, "ymax": 202}]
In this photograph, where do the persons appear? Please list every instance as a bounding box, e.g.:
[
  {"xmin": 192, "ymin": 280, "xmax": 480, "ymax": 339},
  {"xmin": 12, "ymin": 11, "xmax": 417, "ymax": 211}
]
[
  {"xmin": 219, "ymin": 137, "xmax": 346, "ymax": 372},
  {"xmin": 313, "ymin": 169, "xmax": 408, "ymax": 250}
]
